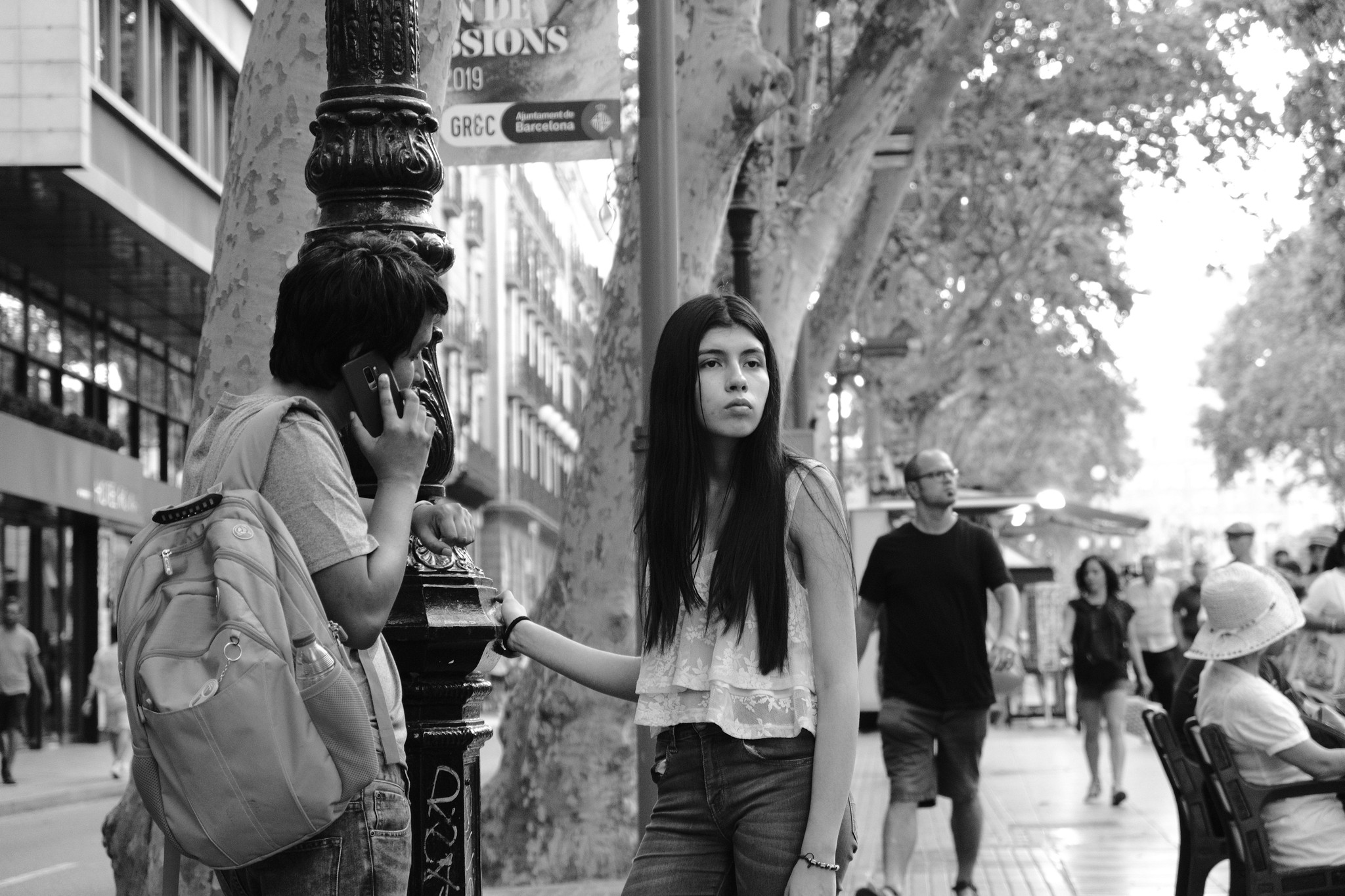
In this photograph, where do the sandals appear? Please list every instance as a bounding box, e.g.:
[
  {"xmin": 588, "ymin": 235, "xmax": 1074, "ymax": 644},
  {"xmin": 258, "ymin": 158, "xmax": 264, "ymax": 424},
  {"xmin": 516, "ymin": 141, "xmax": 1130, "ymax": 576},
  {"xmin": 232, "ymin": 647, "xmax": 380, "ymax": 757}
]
[
  {"xmin": 952, "ymin": 880, "xmax": 979, "ymax": 896},
  {"xmin": 854, "ymin": 882, "xmax": 901, "ymax": 896}
]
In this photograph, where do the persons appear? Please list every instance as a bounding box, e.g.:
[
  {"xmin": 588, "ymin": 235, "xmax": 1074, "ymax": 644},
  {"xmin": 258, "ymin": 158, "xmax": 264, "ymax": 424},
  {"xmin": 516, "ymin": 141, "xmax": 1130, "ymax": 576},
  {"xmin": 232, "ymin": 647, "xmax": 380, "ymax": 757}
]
[
  {"xmin": 1060, "ymin": 520, "xmax": 1345, "ymax": 869},
  {"xmin": 81, "ymin": 623, "xmax": 133, "ymax": 779},
  {"xmin": 854, "ymin": 450, "xmax": 1020, "ymax": 896},
  {"xmin": 0, "ymin": 596, "xmax": 51, "ymax": 784},
  {"xmin": 184, "ymin": 230, "xmax": 475, "ymax": 896},
  {"xmin": 496, "ymin": 294, "xmax": 858, "ymax": 896}
]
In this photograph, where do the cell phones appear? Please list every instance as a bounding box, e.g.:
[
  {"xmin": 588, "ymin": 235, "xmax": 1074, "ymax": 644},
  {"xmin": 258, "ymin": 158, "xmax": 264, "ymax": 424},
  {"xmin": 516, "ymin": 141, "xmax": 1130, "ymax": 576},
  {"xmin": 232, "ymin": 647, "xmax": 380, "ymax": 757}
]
[{"xmin": 342, "ymin": 349, "xmax": 405, "ymax": 438}]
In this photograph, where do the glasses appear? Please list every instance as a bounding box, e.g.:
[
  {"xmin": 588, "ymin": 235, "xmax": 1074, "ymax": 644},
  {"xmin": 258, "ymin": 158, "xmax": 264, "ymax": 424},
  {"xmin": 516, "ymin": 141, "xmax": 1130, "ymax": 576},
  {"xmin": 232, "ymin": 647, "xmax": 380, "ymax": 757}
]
[{"xmin": 913, "ymin": 467, "xmax": 961, "ymax": 480}]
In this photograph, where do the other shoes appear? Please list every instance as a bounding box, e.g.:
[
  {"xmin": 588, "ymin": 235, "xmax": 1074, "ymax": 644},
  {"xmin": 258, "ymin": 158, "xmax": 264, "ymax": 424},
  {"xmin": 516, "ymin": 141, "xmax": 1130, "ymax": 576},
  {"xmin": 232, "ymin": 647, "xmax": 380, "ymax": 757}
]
[
  {"xmin": 1088, "ymin": 783, "xmax": 1101, "ymax": 798},
  {"xmin": 1112, "ymin": 791, "xmax": 1126, "ymax": 805}
]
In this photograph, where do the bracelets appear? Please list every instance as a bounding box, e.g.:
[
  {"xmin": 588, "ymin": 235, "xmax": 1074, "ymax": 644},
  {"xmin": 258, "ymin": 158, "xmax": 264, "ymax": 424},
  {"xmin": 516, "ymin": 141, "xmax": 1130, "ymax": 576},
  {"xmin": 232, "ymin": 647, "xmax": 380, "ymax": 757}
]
[
  {"xmin": 503, "ymin": 615, "xmax": 531, "ymax": 653},
  {"xmin": 413, "ymin": 498, "xmax": 434, "ymax": 511},
  {"xmin": 799, "ymin": 853, "xmax": 840, "ymax": 871},
  {"xmin": 85, "ymin": 698, "xmax": 93, "ymax": 702}
]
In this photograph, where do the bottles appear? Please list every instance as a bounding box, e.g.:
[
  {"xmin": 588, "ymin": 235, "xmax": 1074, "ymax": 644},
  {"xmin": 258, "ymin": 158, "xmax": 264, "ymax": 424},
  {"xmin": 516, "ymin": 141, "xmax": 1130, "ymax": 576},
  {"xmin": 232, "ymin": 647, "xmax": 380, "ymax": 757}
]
[{"xmin": 292, "ymin": 629, "xmax": 335, "ymax": 691}]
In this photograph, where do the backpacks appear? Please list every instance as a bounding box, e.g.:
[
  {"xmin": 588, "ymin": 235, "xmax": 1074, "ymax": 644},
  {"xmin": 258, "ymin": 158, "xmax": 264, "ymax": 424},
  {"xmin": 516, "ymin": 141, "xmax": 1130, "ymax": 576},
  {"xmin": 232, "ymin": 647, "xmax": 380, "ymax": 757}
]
[{"xmin": 112, "ymin": 396, "xmax": 400, "ymax": 896}]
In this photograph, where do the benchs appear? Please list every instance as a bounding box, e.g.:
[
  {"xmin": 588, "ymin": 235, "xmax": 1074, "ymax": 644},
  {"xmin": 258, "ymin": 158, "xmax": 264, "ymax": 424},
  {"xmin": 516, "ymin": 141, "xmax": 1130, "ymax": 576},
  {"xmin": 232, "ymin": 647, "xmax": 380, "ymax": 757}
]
[{"xmin": 1134, "ymin": 696, "xmax": 1345, "ymax": 896}]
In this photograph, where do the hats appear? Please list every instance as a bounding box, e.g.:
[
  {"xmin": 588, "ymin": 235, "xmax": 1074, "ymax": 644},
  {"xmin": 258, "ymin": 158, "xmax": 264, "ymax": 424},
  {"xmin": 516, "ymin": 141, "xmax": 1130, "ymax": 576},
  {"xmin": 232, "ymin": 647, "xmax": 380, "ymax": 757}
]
[
  {"xmin": 1306, "ymin": 527, "xmax": 1338, "ymax": 547},
  {"xmin": 1183, "ymin": 560, "xmax": 1306, "ymax": 659},
  {"xmin": 1225, "ymin": 521, "xmax": 1255, "ymax": 535}
]
